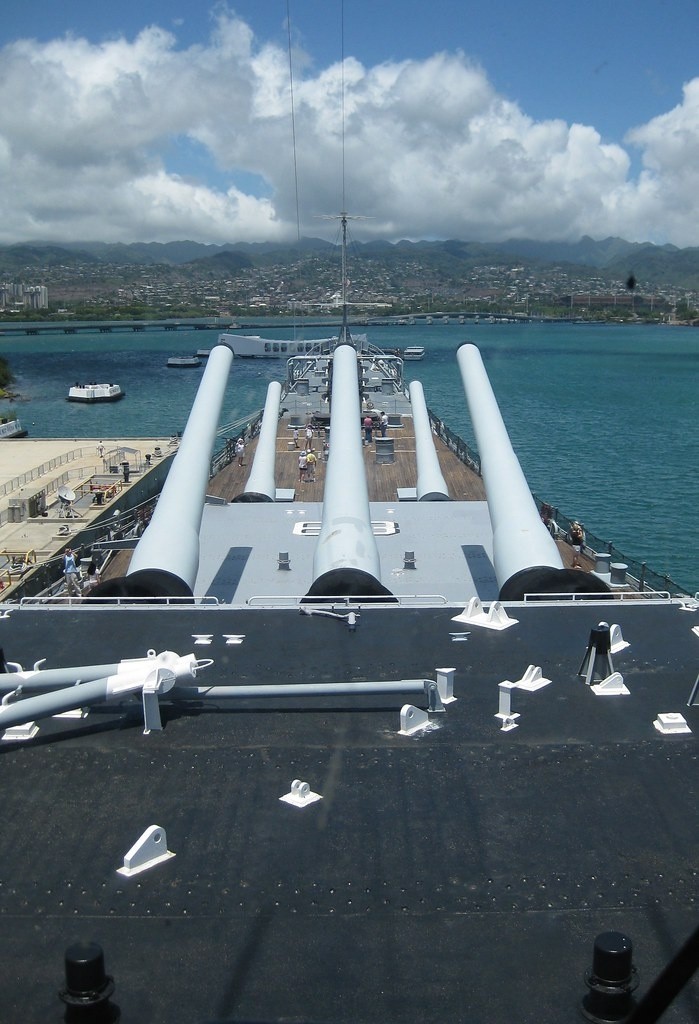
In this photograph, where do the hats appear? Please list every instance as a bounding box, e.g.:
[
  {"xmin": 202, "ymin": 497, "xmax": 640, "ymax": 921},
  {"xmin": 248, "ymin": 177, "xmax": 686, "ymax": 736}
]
[
  {"xmin": 307, "ymin": 449, "xmax": 311, "ymax": 453},
  {"xmin": 307, "ymin": 423, "xmax": 312, "ymax": 427},
  {"xmin": 299, "ymin": 450, "xmax": 306, "ymax": 456},
  {"xmin": 238, "ymin": 438, "xmax": 243, "ymax": 442}
]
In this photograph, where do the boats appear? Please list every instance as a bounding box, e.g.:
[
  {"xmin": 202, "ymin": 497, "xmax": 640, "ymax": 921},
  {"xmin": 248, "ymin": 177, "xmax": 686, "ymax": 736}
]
[
  {"xmin": 404, "ymin": 346, "xmax": 426, "ymax": 361},
  {"xmin": 0, "ymin": 0, "xmax": 698, "ymax": 1022},
  {"xmin": 196, "ymin": 332, "xmax": 369, "ymax": 358}
]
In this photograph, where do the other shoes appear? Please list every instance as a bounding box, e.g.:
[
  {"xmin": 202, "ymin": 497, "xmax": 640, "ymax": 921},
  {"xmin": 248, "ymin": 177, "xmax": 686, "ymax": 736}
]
[
  {"xmin": 298, "ymin": 480, "xmax": 301, "ymax": 482},
  {"xmin": 239, "ymin": 465, "xmax": 241, "ymax": 467},
  {"xmin": 295, "ymin": 446, "xmax": 300, "ymax": 448},
  {"xmin": 307, "ymin": 480, "xmax": 310, "ymax": 483},
  {"xmin": 300, "ymin": 481, "xmax": 305, "ymax": 484},
  {"xmin": 240, "ymin": 464, "xmax": 243, "ymax": 466},
  {"xmin": 314, "ymin": 480, "xmax": 316, "ymax": 483}
]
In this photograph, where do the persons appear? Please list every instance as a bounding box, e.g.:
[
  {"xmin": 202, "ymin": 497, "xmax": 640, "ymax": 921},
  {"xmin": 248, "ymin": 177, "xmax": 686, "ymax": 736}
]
[
  {"xmin": 567, "ymin": 521, "xmax": 584, "ymax": 568},
  {"xmin": 97, "ymin": 441, "xmax": 105, "ymax": 457},
  {"xmin": 73, "ymin": 551, "xmax": 82, "ymax": 580},
  {"xmin": 545, "ymin": 515, "xmax": 559, "ymax": 542},
  {"xmin": 305, "ymin": 425, "xmax": 313, "ymax": 449},
  {"xmin": 236, "ymin": 438, "xmax": 245, "ymax": 467},
  {"xmin": 299, "ymin": 451, "xmax": 313, "ymax": 483},
  {"xmin": 303, "ymin": 449, "xmax": 317, "ymax": 483},
  {"xmin": 86, "ymin": 561, "xmax": 96, "ymax": 580},
  {"xmin": 63, "ymin": 548, "xmax": 82, "ymax": 597},
  {"xmin": 364, "ymin": 416, "xmax": 373, "ymax": 443},
  {"xmin": 379, "ymin": 411, "xmax": 388, "ymax": 437},
  {"xmin": 293, "ymin": 428, "xmax": 300, "ymax": 448}
]
